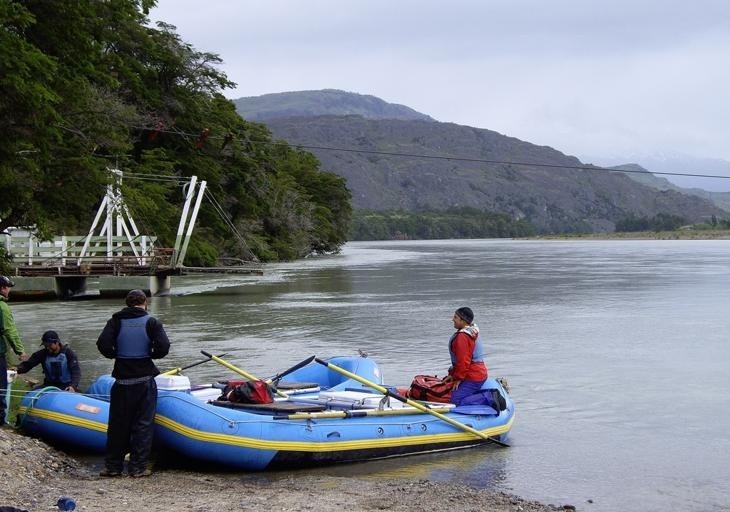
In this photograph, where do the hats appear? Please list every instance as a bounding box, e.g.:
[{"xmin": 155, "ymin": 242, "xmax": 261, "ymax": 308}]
[
  {"xmin": 40, "ymin": 330, "xmax": 60, "ymax": 346},
  {"xmin": 0, "ymin": 276, "xmax": 16, "ymax": 287},
  {"xmin": 455, "ymin": 307, "xmax": 473, "ymax": 324}
]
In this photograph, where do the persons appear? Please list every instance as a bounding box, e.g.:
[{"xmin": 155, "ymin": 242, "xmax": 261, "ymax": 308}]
[
  {"xmin": 443, "ymin": 307, "xmax": 507, "ymax": 417},
  {"xmin": 0, "ymin": 275, "xmax": 27, "ymax": 430},
  {"xmin": 96, "ymin": 289, "xmax": 170, "ymax": 477},
  {"xmin": 7, "ymin": 330, "xmax": 83, "ymax": 394}
]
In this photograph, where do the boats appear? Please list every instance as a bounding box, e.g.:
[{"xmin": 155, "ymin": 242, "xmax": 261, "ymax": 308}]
[
  {"xmin": 13, "ymin": 350, "xmax": 387, "ymax": 462},
  {"xmin": 152, "ymin": 365, "xmax": 527, "ymax": 474}
]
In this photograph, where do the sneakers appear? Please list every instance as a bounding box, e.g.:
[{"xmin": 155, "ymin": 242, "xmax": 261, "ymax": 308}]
[
  {"xmin": 132, "ymin": 469, "xmax": 152, "ymax": 477},
  {"xmin": 492, "ymin": 389, "xmax": 506, "ymax": 411},
  {"xmin": 491, "ymin": 391, "xmax": 500, "ymax": 416},
  {"xmin": 100, "ymin": 467, "xmax": 121, "ymax": 477}
]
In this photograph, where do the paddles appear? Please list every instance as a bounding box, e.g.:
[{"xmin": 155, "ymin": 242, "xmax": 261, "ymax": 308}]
[
  {"xmin": 265, "ymin": 356, "xmax": 317, "ymax": 382},
  {"xmin": 159, "ymin": 352, "xmax": 226, "ymax": 375},
  {"xmin": 314, "ymin": 358, "xmax": 510, "ymax": 448},
  {"xmin": 273, "ymin": 405, "xmax": 495, "ymax": 420}
]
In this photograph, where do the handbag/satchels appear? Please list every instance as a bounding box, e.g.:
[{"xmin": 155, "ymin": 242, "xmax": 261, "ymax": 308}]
[
  {"xmin": 409, "ymin": 375, "xmax": 456, "ymax": 403},
  {"xmin": 218, "ymin": 380, "xmax": 274, "ymax": 404}
]
